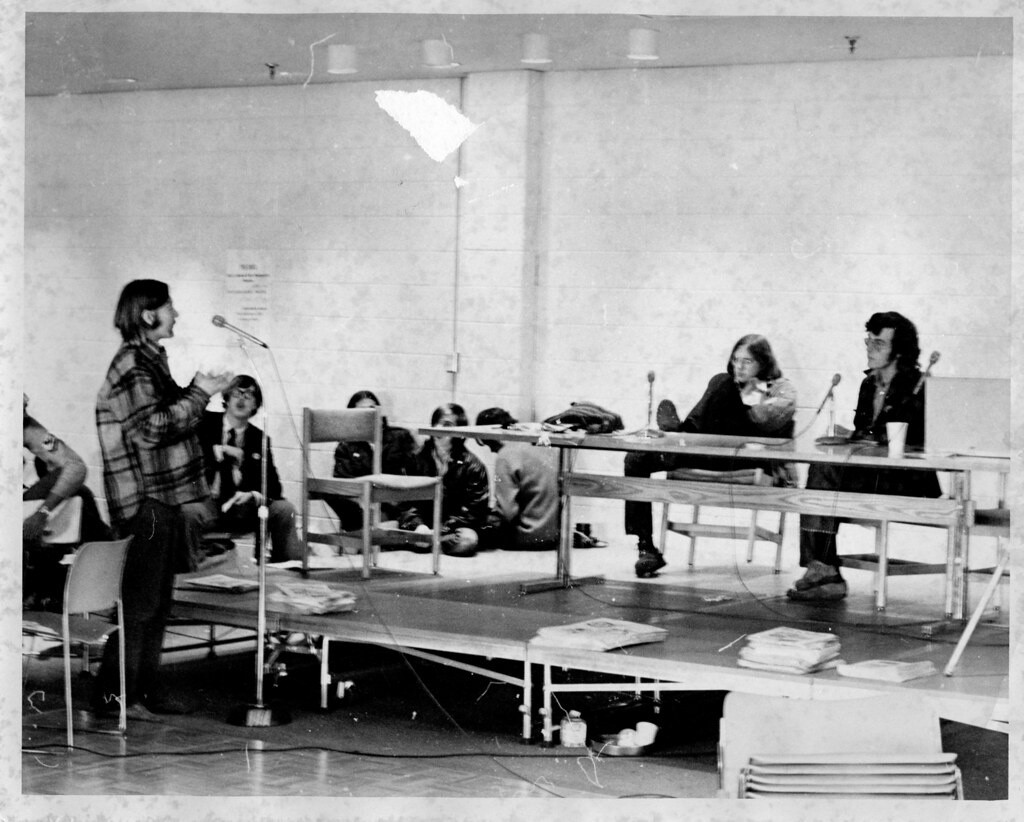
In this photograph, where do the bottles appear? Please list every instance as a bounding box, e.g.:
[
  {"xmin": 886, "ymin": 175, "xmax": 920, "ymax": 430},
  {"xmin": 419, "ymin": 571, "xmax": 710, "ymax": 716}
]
[{"xmin": 559, "ymin": 708, "xmax": 590, "ymax": 750}]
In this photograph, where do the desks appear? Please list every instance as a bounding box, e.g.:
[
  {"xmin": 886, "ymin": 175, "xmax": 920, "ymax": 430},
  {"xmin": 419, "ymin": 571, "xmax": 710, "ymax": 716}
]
[{"xmin": 412, "ymin": 422, "xmax": 1011, "ymax": 627}]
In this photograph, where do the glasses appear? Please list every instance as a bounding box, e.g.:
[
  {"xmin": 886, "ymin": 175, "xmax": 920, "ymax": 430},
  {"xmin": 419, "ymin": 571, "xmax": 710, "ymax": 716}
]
[
  {"xmin": 231, "ymin": 390, "xmax": 255, "ymax": 400},
  {"xmin": 865, "ymin": 338, "xmax": 890, "ymax": 351},
  {"xmin": 732, "ymin": 357, "xmax": 755, "ymax": 364}
]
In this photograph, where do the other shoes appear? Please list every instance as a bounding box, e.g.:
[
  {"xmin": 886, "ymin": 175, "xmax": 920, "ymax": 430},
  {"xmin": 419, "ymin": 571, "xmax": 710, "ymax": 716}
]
[
  {"xmin": 106, "ymin": 695, "xmax": 194, "ymax": 724},
  {"xmin": 656, "ymin": 400, "xmax": 680, "ymax": 431},
  {"xmin": 635, "ymin": 542, "xmax": 667, "ymax": 575},
  {"xmin": 787, "ymin": 561, "xmax": 847, "ymax": 601}
]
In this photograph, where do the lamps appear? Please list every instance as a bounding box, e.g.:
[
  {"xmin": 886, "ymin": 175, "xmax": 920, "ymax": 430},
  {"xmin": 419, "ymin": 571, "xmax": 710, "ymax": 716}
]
[{"xmin": 328, "ymin": 17, "xmax": 658, "ymax": 74}]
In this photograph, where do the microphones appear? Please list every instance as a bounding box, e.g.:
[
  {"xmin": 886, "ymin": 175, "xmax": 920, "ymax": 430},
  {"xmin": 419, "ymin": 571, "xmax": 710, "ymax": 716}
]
[
  {"xmin": 211, "ymin": 314, "xmax": 268, "ymax": 349},
  {"xmin": 913, "ymin": 351, "xmax": 940, "ymax": 394},
  {"xmin": 647, "ymin": 370, "xmax": 655, "ymax": 412},
  {"xmin": 817, "ymin": 374, "xmax": 840, "ymax": 413}
]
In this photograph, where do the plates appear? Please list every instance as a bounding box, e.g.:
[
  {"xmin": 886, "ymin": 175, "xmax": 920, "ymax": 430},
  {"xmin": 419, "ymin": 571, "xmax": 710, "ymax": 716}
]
[{"xmin": 592, "ymin": 734, "xmax": 649, "ymax": 755}]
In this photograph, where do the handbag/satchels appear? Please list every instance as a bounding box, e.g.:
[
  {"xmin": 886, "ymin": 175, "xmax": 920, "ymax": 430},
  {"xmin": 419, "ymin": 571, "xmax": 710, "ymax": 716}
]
[{"xmin": 544, "ymin": 403, "xmax": 625, "ymax": 435}]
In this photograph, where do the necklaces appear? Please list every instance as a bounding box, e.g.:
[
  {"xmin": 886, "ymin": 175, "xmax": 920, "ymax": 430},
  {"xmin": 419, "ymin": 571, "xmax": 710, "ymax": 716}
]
[{"xmin": 877, "ymin": 390, "xmax": 884, "ymax": 397}]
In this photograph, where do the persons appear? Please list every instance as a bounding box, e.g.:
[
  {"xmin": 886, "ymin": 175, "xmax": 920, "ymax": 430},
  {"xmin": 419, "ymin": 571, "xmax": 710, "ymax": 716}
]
[
  {"xmin": 23, "ymin": 393, "xmax": 112, "ymax": 609},
  {"xmin": 788, "ymin": 311, "xmax": 942, "ymax": 602},
  {"xmin": 88, "ymin": 277, "xmax": 237, "ymax": 724},
  {"xmin": 332, "ymin": 392, "xmax": 420, "ymax": 551},
  {"xmin": 475, "ymin": 407, "xmax": 561, "ymax": 551},
  {"xmin": 201, "ymin": 375, "xmax": 297, "ymax": 564},
  {"xmin": 397, "ymin": 403, "xmax": 490, "ymax": 557},
  {"xmin": 623, "ymin": 334, "xmax": 798, "ymax": 575}
]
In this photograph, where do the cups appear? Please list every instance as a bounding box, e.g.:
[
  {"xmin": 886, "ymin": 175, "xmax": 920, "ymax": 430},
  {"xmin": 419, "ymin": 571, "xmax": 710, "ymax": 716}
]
[
  {"xmin": 886, "ymin": 422, "xmax": 908, "ymax": 454},
  {"xmin": 618, "ymin": 721, "xmax": 660, "ymax": 746}
]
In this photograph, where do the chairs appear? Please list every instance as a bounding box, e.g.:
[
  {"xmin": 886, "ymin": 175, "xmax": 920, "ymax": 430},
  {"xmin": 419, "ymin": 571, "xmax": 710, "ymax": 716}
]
[
  {"xmin": 299, "ymin": 406, "xmax": 442, "ymax": 580},
  {"xmin": 656, "ymin": 375, "xmax": 1014, "ymax": 576},
  {"xmin": 717, "ymin": 690, "xmax": 963, "ymax": 801},
  {"xmin": 22, "ymin": 533, "xmax": 134, "ymax": 752}
]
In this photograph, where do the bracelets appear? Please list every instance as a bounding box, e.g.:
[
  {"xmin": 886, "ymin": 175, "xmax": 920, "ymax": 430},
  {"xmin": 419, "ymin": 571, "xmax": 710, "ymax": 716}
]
[{"xmin": 36, "ymin": 505, "xmax": 50, "ymax": 516}]
